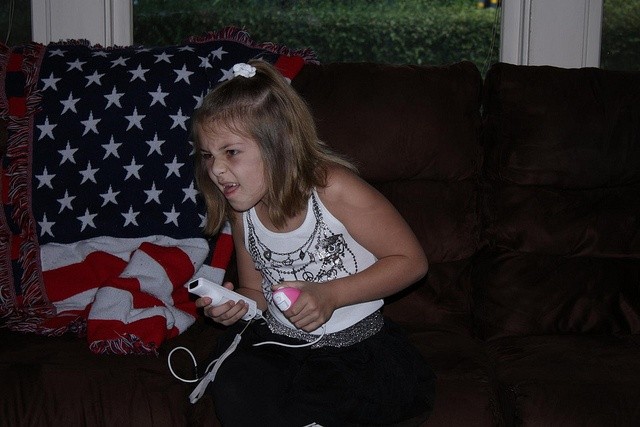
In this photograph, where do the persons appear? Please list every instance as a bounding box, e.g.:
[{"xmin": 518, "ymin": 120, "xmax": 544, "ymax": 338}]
[{"xmin": 192, "ymin": 60, "xmax": 435, "ymax": 426}]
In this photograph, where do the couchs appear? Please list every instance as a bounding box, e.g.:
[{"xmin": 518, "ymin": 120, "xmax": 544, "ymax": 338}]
[{"xmin": 1, "ymin": 47, "xmax": 636, "ymax": 426}]
